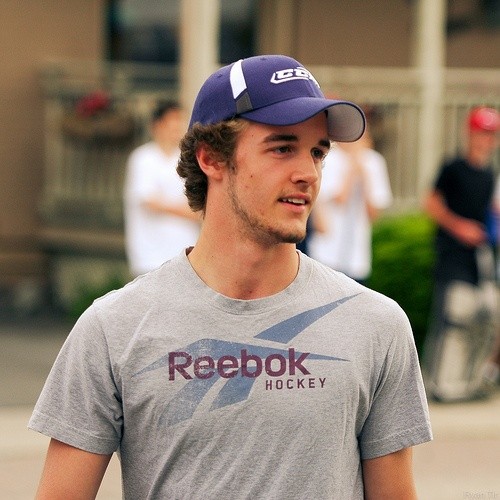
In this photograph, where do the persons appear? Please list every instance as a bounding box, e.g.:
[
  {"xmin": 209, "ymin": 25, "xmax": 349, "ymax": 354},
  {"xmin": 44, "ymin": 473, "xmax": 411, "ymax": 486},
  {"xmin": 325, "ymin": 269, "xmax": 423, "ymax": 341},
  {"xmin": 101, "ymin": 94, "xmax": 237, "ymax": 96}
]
[
  {"xmin": 306, "ymin": 100, "xmax": 393, "ymax": 280},
  {"xmin": 26, "ymin": 55, "xmax": 432, "ymax": 500},
  {"xmin": 126, "ymin": 98, "xmax": 203, "ymax": 283},
  {"xmin": 424, "ymin": 105, "xmax": 500, "ymax": 345}
]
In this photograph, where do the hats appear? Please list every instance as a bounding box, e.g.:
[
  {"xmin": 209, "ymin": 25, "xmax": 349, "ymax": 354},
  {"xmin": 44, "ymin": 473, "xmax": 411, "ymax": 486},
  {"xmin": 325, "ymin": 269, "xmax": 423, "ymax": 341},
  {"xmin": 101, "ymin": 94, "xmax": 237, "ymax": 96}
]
[
  {"xmin": 469, "ymin": 106, "xmax": 499, "ymax": 132},
  {"xmin": 187, "ymin": 54, "xmax": 366, "ymax": 143}
]
